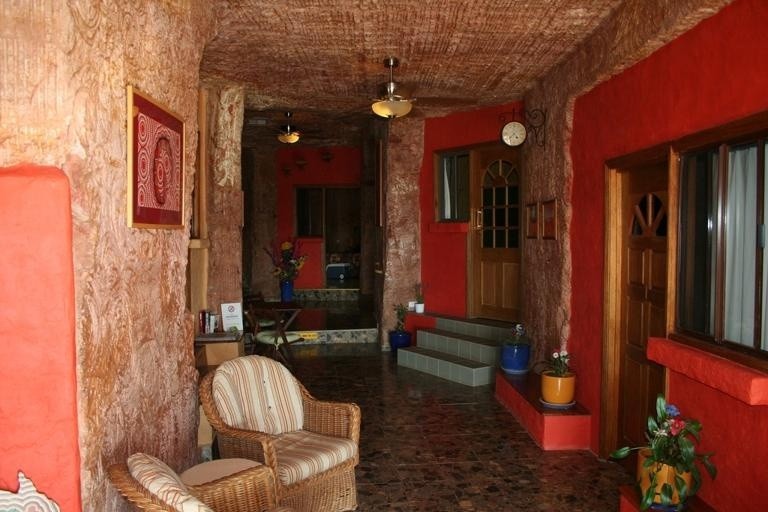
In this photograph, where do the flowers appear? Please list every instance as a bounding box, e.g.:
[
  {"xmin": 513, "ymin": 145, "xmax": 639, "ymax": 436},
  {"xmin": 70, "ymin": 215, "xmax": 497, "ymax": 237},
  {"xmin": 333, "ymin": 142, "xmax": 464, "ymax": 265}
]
[
  {"xmin": 263, "ymin": 237, "xmax": 306, "ymax": 282},
  {"xmin": 608, "ymin": 393, "xmax": 718, "ymax": 512},
  {"xmin": 532, "ymin": 346, "xmax": 576, "ymax": 376},
  {"xmin": 503, "ymin": 328, "xmax": 530, "ymax": 346}
]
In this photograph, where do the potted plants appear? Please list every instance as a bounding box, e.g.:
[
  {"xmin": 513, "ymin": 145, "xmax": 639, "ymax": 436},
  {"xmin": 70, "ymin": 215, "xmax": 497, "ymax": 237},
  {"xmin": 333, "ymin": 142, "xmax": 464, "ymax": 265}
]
[{"xmin": 389, "ymin": 301, "xmax": 412, "ymax": 351}]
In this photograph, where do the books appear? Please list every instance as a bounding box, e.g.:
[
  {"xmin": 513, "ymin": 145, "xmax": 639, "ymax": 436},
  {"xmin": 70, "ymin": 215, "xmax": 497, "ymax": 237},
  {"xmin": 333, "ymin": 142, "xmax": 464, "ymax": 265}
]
[
  {"xmin": 198, "ymin": 309, "xmax": 205, "ymax": 334},
  {"xmin": 220, "ymin": 301, "xmax": 244, "ymax": 332},
  {"xmin": 204, "ymin": 311, "xmax": 210, "ymax": 334},
  {"xmin": 209, "ymin": 313, "xmax": 219, "ymax": 334}
]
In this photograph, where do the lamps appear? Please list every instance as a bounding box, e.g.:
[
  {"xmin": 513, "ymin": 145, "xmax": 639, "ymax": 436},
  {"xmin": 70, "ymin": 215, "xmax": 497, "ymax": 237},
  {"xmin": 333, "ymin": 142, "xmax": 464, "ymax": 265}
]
[
  {"xmin": 276, "ymin": 111, "xmax": 301, "ymax": 145},
  {"xmin": 371, "ymin": 56, "xmax": 413, "ymax": 120}
]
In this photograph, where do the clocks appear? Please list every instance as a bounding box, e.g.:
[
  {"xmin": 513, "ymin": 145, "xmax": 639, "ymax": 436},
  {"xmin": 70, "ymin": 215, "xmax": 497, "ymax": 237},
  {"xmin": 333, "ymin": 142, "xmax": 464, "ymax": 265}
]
[{"xmin": 501, "ymin": 121, "xmax": 527, "ymax": 146}]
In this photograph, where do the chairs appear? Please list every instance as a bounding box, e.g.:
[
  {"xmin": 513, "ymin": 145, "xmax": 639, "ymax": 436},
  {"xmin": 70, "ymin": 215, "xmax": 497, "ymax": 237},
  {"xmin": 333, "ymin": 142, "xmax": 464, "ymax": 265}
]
[{"xmin": 245, "ymin": 304, "xmax": 304, "ymax": 375}]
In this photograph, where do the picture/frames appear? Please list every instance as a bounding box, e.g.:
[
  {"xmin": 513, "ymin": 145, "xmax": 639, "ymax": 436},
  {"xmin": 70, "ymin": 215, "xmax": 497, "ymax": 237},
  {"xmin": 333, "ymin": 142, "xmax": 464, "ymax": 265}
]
[{"xmin": 125, "ymin": 83, "xmax": 188, "ymax": 232}]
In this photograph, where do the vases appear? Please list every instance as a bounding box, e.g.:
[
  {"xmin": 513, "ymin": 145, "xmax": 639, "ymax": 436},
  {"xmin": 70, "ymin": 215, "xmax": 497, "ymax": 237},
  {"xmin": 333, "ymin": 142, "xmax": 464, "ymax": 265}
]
[
  {"xmin": 280, "ymin": 279, "xmax": 294, "ymax": 302},
  {"xmin": 501, "ymin": 344, "xmax": 531, "ymax": 374},
  {"xmin": 538, "ymin": 372, "xmax": 576, "ymax": 410},
  {"xmin": 636, "ymin": 447, "xmax": 693, "ymax": 511}
]
